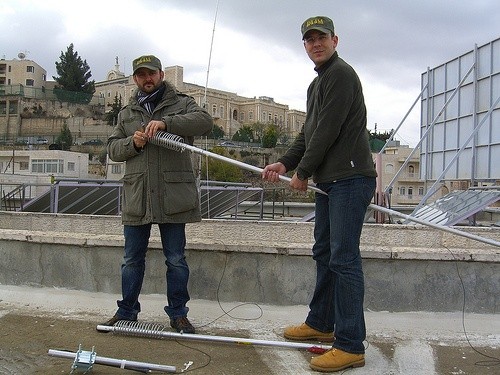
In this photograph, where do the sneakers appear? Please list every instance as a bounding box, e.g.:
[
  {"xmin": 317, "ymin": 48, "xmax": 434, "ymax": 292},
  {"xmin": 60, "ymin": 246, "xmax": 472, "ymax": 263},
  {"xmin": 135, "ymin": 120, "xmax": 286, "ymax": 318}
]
[
  {"xmin": 170, "ymin": 315, "xmax": 195, "ymax": 335},
  {"xmin": 310, "ymin": 347, "xmax": 365, "ymax": 372},
  {"xmin": 285, "ymin": 323, "xmax": 334, "ymax": 343},
  {"xmin": 96, "ymin": 312, "xmax": 137, "ymax": 333}
]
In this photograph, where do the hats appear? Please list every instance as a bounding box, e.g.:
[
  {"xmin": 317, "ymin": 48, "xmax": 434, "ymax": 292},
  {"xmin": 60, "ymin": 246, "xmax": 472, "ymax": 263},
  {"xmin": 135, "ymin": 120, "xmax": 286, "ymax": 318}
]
[
  {"xmin": 301, "ymin": 16, "xmax": 334, "ymax": 39},
  {"xmin": 133, "ymin": 56, "xmax": 161, "ymax": 74}
]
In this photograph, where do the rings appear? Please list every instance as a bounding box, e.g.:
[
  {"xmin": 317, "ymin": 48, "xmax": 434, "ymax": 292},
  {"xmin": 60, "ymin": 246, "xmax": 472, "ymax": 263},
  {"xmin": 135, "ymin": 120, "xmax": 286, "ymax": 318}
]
[{"xmin": 141, "ymin": 137, "xmax": 144, "ymax": 141}]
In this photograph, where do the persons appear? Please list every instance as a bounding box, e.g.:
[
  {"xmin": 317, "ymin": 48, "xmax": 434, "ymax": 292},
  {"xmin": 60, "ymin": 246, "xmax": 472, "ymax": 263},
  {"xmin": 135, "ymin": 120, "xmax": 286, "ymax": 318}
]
[
  {"xmin": 96, "ymin": 55, "xmax": 213, "ymax": 334},
  {"xmin": 262, "ymin": 16, "xmax": 379, "ymax": 372}
]
